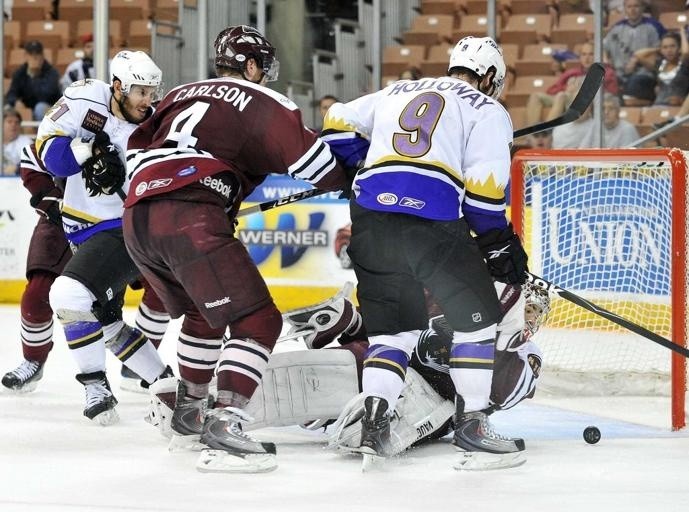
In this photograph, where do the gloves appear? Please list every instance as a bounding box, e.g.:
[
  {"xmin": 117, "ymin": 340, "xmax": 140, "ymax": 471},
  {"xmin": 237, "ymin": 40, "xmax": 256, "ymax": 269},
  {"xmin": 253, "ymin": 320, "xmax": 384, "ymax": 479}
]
[
  {"xmin": 475, "ymin": 223, "xmax": 528, "ymax": 284},
  {"xmin": 83, "ymin": 132, "xmax": 126, "ymax": 195}
]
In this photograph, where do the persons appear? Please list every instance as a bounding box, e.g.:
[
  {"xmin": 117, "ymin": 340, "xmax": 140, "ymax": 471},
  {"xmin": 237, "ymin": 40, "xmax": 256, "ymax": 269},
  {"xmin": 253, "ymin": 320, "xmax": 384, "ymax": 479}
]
[
  {"xmin": 120, "ymin": 25, "xmax": 350, "ymax": 474},
  {"xmin": 59, "ymin": 34, "xmax": 96, "ymax": 91},
  {"xmin": 2, "ymin": 142, "xmax": 170, "ymax": 395},
  {"xmin": 2, "ymin": 40, "xmax": 62, "ymax": 123},
  {"xmin": 2, "ymin": 110, "xmax": 37, "ymax": 176},
  {"xmin": 321, "ymin": 36, "xmax": 550, "ymax": 475},
  {"xmin": 526, "ymin": 1, "xmax": 688, "ymax": 152},
  {"xmin": 37, "ymin": 52, "xmax": 165, "ymax": 421}
]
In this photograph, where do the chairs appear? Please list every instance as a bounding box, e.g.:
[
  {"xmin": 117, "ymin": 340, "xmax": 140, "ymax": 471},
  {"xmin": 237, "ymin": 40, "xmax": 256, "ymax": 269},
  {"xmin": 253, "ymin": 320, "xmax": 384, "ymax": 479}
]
[
  {"xmin": 374, "ymin": 1, "xmax": 689, "ymax": 148},
  {"xmin": 1, "ymin": 1, "xmax": 199, "ymax": 178}
]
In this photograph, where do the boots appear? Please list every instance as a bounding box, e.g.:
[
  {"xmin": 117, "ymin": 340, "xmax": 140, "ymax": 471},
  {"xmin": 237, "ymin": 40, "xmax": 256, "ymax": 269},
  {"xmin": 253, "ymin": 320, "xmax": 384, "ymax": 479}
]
[
  {"xmin": 451, "ymin": 394, "xmax": 525, "ymax": 452},
  {"xmin": 76, "ymin": 370, "xmax": 118, "ymax": 419},
  {"xmin": 2, "ymin": 343, "xmax": 54, "ymax": 387},
  {"xmin": 359, "ymin": 396, "xmax": 391, "ymax": 456},
  {"xmin": 121, "ymin": 365, "xmax": 276, "ymax": 453}
]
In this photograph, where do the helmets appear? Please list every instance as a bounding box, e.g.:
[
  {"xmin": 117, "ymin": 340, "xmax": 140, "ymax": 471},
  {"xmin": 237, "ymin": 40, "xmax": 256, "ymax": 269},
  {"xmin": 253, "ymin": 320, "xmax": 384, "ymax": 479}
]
[
  {"xmin": 214, "ymin": 25, "xmax": 280, "ymax": 82},
  {"xmin": 448, "ymin": 35, "xmax": 507, "ymax": 100},
  {"xmin": 521, "ymin": 281, "xmax": 551, "ymax": 334},
  {"xmin": 111, "ymin": 50, "xmax": 162, "ymax": 94}
]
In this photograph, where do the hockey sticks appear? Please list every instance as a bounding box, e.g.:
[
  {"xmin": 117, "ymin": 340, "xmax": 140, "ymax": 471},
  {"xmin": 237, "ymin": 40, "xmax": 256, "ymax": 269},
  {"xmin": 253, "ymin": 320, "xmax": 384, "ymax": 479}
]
[{"xmin": 238, "ymin": 64, "xmax": 605, "ymax": 221}]
